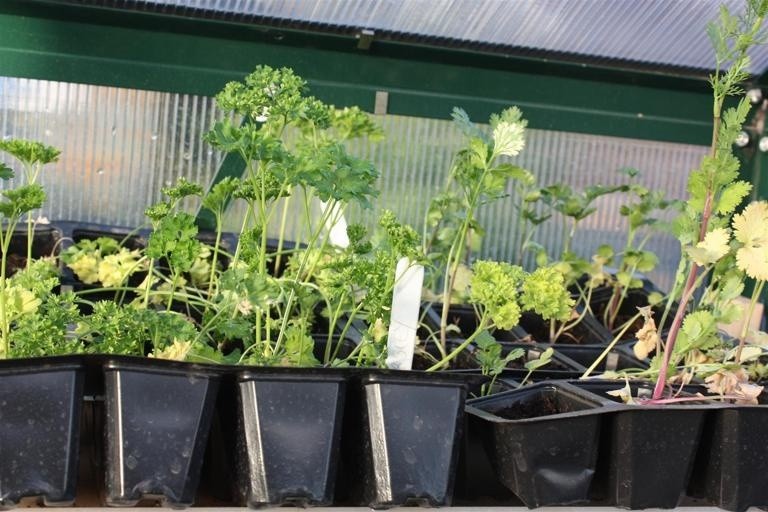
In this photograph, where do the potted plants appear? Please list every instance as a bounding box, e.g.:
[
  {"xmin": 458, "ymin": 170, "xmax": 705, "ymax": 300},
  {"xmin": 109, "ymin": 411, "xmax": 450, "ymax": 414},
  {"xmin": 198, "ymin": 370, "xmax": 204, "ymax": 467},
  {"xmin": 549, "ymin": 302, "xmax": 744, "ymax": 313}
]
[{"xmin": 0, "ymin": 0, "xmax": 768, "ymax": 512}]
